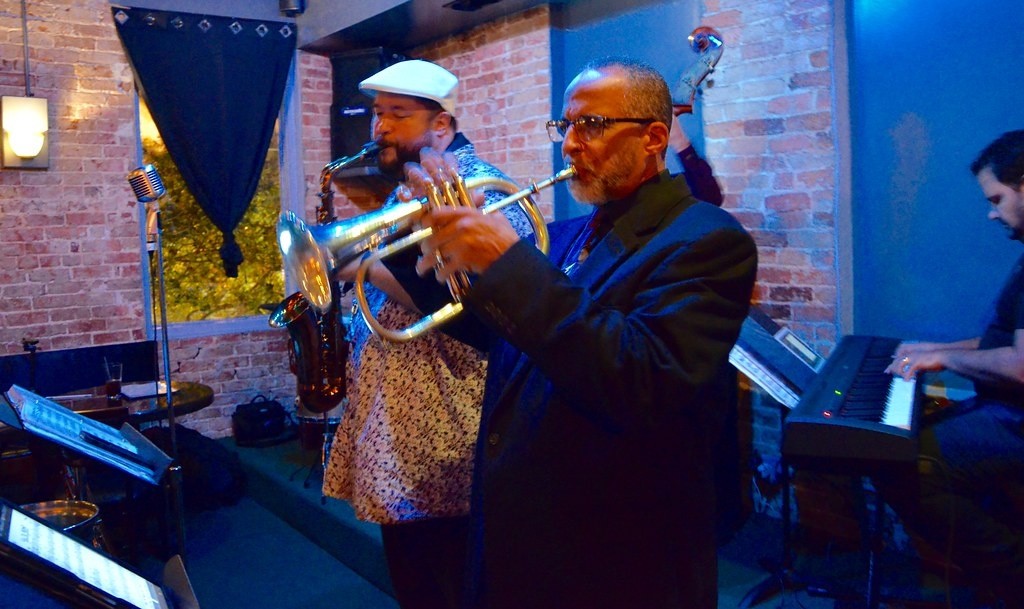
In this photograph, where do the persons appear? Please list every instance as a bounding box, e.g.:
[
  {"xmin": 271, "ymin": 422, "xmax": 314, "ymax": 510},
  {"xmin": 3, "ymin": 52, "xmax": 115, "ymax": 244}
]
[
  {"xmin": 395, "ymin": 58, "xmax": 758, "ymax": 609},
  {"xmin": 322, "ymin": 61, "xmax": 540, "ymax": 609},
  {"xmin": 883, "ymin": 127, "xmax": 1024, "ymax": 609}
]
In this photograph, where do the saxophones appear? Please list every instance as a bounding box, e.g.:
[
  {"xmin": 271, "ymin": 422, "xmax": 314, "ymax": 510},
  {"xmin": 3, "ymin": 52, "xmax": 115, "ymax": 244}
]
[{"xmin": 267, "ymin": 140, "xmax": 388, "ymax": 415}]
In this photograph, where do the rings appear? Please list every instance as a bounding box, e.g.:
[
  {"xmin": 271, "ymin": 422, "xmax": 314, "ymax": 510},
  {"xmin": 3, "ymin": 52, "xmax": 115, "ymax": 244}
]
[{"xmin": 902, "ymin": 356, "xmax": 909, "ymax": 363}]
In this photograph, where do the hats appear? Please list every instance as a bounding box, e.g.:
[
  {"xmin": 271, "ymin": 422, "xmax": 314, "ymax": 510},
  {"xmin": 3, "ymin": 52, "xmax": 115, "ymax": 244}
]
[{"xmin": 359, "ymin": 59, "xmax": 458, "ymax": 118}]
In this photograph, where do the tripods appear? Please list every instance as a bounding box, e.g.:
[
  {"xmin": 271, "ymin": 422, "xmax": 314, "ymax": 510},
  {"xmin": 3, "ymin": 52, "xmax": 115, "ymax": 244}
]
[
  {"xmin": 729, "ymin": 308, "xmax": 853, "ymax": 609},
  {"xmin": 304, "ymin": 410, "xmax": 334, "ymax": 504}
]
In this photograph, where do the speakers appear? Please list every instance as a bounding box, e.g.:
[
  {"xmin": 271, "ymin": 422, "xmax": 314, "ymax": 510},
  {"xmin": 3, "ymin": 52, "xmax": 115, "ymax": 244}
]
[{"xmin": 328, "ymin": 47, "xmax": 413, "ymax": 170}]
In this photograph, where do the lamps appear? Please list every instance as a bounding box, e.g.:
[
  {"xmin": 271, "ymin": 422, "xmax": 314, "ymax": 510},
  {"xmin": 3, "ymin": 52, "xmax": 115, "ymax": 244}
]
[{"xmin": 1, "ymin": 96, "xmax": 50, "ymax": 170}]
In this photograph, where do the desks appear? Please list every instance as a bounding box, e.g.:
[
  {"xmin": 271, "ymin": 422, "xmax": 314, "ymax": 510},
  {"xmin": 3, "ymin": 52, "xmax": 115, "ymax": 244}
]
[{"xmin": 60, "ymin": 381, "xmax": 214, "ymax": 558}]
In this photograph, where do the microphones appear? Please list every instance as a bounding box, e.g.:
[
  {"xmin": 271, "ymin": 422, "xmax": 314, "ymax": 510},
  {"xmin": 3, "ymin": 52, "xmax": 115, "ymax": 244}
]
[{"xmin": 127, "ymin": 163, "xmax": 166, "ymax": 258}]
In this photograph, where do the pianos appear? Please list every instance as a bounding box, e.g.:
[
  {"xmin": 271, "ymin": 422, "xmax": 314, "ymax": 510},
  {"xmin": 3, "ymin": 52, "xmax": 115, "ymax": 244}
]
[{"xmin": 781, "ymin": 333, "xmax": 924, "ymax": 463}]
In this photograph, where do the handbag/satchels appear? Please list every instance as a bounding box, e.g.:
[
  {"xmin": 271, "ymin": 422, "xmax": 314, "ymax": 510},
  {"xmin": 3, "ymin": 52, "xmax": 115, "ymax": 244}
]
[{"xmin": 231, "ymin": 394, "xmax": 298, "ymax": 448}]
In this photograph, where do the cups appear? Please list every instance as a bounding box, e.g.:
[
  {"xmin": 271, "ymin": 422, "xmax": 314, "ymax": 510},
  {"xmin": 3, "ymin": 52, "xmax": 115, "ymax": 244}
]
[{"xmin": 102, "ymin": 362, "xmax": 123, "ymax": 401}]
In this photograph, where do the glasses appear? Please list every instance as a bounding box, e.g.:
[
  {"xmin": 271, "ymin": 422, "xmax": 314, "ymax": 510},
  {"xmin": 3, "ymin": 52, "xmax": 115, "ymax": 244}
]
[{"xmin": 545, "ymin": 113, "xmax": 657, "ymax": 144}]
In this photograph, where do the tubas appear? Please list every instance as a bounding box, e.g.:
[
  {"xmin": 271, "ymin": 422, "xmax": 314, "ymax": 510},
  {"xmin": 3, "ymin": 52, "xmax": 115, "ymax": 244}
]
[{"xmin": 277, "ymin": 164, "xmax": 578, "ymax": 345}]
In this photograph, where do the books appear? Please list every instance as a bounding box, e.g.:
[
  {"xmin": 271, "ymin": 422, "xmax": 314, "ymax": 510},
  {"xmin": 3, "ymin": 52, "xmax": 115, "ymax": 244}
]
[
  {"xmin": 9, "ymin": 385, "xmax": 156, "ymax": 477},
  {"xmin": 729, "ymin": 306, "xmax": 827, "ymax": 409}
]
